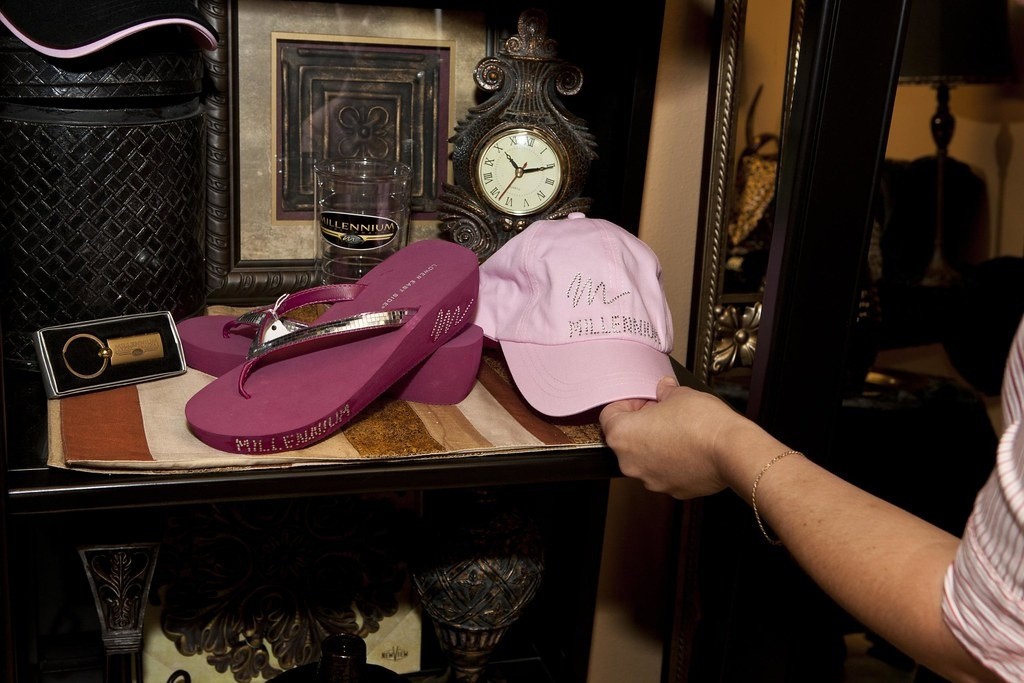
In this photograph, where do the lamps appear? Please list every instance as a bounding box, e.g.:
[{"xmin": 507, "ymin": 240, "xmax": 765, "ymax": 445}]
[{"xmin": 897, "ymin": 0, "xmax": 1016, "ymax": 289}]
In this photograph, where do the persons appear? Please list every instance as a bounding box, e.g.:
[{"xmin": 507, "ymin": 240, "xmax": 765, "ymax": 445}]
[{"xmin": 597, "ymin": 311, "xmax": 1024, "ymax": 683}]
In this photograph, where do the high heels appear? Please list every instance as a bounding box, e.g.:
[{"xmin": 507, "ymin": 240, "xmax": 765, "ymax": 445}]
[
  {"xmin": 186, "ymin": 238, "xmax": 478, "ymax": 456},
  {"xmin": 176, "ymin": 303, "xmax": 484, "ymax": 406}
]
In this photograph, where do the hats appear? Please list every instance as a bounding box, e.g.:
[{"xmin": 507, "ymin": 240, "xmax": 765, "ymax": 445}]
[{"xmin": 471, "ymin": 212, "xmax": 681, "ymax": 418}]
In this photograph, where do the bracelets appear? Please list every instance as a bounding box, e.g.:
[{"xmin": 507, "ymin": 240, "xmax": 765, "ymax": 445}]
[{"xmin": 751, "ymin": 451, "xmax": 805, "ymax": 546}]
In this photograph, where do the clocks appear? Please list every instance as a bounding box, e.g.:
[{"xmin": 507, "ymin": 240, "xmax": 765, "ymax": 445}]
[{"xmin": 437, "ymin": 4, "xmax": 603, "ymax": 262}]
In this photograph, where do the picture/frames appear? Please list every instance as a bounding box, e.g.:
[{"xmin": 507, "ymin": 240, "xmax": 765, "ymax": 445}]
[{"xmin": 191, "ymin": 0, "xmax": 517, "ymax": 304}]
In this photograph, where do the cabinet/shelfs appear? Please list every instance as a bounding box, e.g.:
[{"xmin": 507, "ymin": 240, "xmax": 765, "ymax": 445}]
[{"xmin": 0, "ymin": 346, "xmax": 624, "ymax": 683}]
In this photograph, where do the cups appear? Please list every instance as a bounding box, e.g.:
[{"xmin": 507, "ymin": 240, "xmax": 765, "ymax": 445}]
[{"xmin": 313, "ymin": 160, "xmax": 412, "ymax": 290}]
